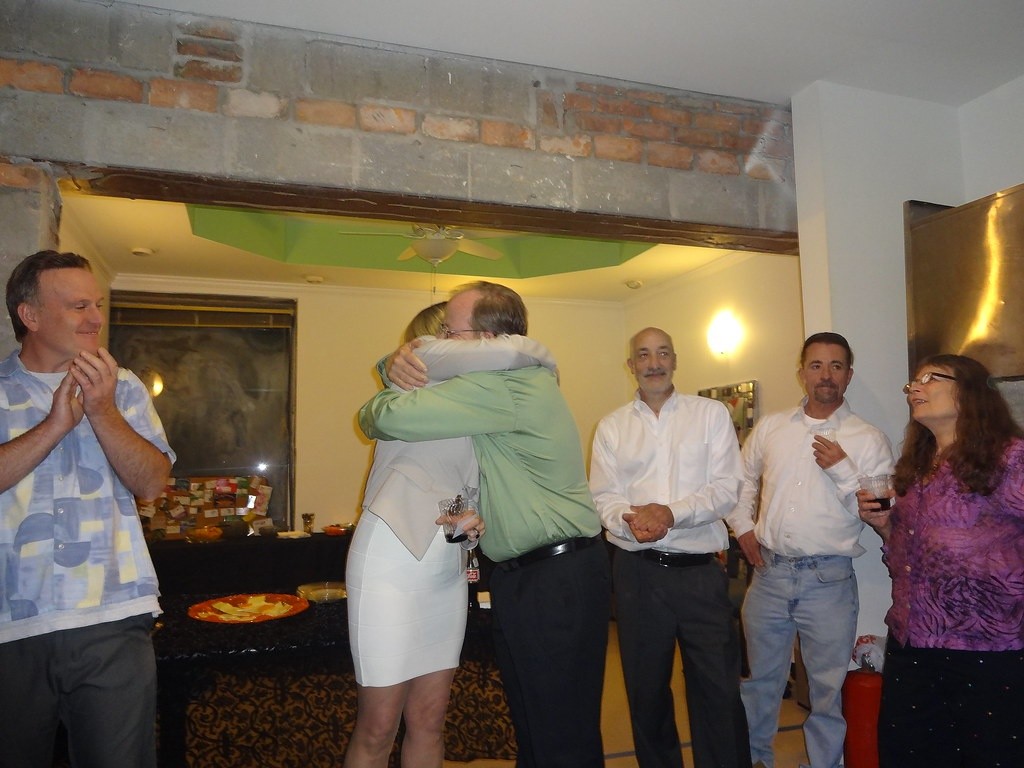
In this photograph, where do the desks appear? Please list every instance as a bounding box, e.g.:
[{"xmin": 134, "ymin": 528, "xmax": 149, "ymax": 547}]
[{"xmin": 150, "ymin": 535, "xmax": 519, "ymax": 768}]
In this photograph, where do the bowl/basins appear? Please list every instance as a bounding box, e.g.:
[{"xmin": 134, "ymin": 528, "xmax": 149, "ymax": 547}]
[{"xmin": 186, "ymin": 527, "xmax": 223, "ymax": 543}]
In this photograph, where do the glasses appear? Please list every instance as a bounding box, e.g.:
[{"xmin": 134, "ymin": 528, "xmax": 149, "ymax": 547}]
[
  {"xmin": 903, "ymin": 372, "xmax": 956, "ymax": 394},
  {"xmin": 439, "ymin": 322, "xmax": 497, "ymax": 339}
]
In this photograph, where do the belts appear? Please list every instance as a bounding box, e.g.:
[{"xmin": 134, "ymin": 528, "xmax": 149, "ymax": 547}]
[
  {"xmin": 637, "ymin": 550, "xmax": 713, "ymax": 568},
  {"xmin": 500, "ymin": 534, "xmax": 601, "ymax": 572}
]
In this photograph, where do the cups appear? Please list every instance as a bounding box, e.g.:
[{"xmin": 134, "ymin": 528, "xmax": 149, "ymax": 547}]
[
  {"xmin": 438, "ymin": 498, "xmax": 468, "ymax": 543},
  {"xmin": 811, "ymin": 428, "xmax": 835, "ymax": 448},
  {"xmin": 858, "ymin": 474, "xmax": 890, "ymax": 511},
  {"xmin": 303, "ymin": 513, "xmax": 315, "ymax": 535}
]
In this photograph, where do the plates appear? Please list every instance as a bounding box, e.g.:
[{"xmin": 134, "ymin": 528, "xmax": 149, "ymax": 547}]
[
  {"xmin": 188, "ymin": 593, "xmax": 310, "ymax": 624},
  {"xmin": 297, "ymin": 582, "xmax": 347, "ymax": 602},
  {"xmin": 321, "ymin": 526, "xmax": 347, "ymax": 536}
]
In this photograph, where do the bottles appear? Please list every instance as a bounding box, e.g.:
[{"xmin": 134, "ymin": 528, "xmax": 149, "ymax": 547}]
[{"xmin": 467, "ymin": 549, "xmax": 480, "ymax": 613}]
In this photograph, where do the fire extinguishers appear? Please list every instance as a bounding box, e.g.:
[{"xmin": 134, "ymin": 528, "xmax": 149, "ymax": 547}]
[{"xmin": 841, "ymin": 652, "xmax": 883, "ymax": 768}]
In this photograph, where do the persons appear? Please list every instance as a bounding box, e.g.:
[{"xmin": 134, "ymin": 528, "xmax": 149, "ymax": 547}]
[
  {"xmin": 589, "ymin": 327, "xmax": 754, "ymax": 768},
  {"xmin": 726, "ymin": 331, "xmax": 898, "ymax": 768},
  {"xmin": 0, "ymin": 249, "xmax": 177, "ymax": 768},
  {"xmin": 855, "ymin": 352, "xmax": 1024, "ymax": 768},
  {"xmin": 344, "ymin": 280, "xmax": 614, "ymax": 768}
]
